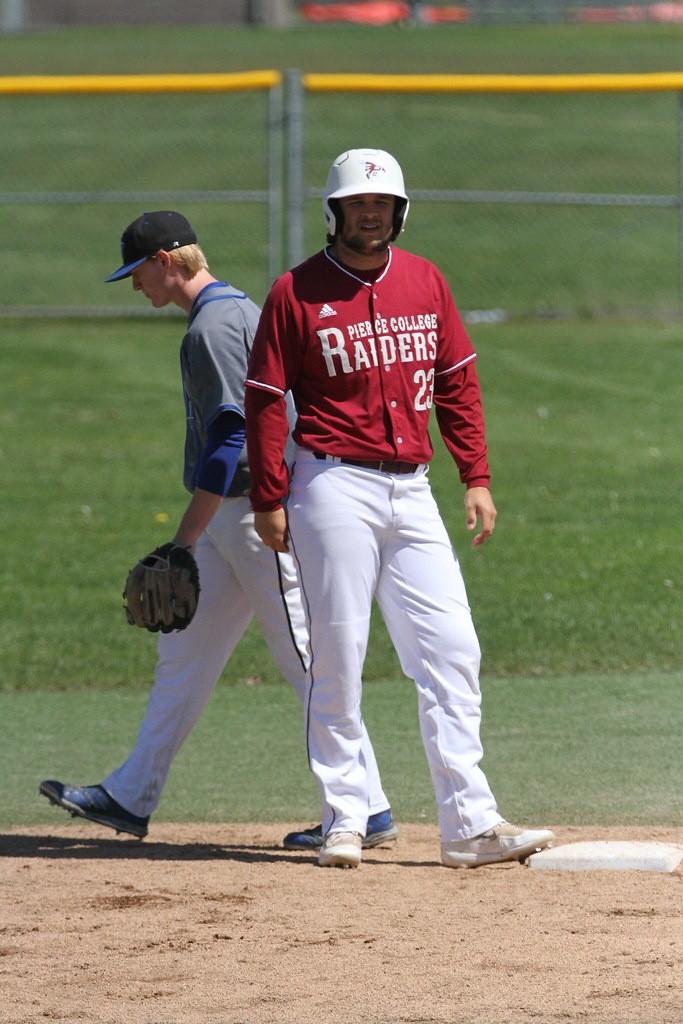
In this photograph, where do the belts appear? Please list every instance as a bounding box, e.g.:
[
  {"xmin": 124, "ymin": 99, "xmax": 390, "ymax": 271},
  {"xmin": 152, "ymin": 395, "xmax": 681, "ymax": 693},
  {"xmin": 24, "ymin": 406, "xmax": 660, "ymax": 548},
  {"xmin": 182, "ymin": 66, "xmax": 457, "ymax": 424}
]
[{"xmin": 313, "ymin": 450, "xmax": 428, "ymax": 475}]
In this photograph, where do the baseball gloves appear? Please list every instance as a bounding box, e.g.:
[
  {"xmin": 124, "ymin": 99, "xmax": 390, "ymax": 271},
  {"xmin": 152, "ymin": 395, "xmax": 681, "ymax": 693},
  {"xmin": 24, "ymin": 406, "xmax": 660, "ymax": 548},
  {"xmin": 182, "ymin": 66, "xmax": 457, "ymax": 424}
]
[{"xmin": 121, "ymin": 542, "xmax": 202, "ymax": 635}]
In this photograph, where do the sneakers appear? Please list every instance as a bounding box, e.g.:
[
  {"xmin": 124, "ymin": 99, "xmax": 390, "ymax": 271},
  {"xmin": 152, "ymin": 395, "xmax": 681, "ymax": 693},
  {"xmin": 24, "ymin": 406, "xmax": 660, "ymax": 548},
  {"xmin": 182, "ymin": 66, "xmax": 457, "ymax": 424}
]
[
  {"xmin": 283, "ymin": 808, "xmax": 399, "ymax": 850},
  {"xmin": 441, "ymin": 820, "xmax": 555, "ymax": 868},
  {"xmin": 319, "ymin": 831, "xmax": 362, "ymax": 869},
  {"xmin": 39, "ymin": 781, "xmax": 151, "ymax": 840}
]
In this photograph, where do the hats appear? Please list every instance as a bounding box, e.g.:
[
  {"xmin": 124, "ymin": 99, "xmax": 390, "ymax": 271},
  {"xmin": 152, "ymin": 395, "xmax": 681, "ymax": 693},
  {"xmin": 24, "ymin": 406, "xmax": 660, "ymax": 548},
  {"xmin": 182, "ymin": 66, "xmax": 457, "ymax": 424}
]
[{"xmin": 104, "ymin": 211, "xmax": 197, "ymax": 284}]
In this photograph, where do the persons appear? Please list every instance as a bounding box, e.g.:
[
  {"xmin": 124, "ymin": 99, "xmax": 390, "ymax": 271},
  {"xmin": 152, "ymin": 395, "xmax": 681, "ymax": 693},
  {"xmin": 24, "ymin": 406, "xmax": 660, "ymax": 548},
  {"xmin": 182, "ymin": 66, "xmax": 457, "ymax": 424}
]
[
  {"xmin": 41, "ymin": 210, "xmax": 398, "ymax": 847},
  {"xmin": 243, "ymin": 149, "xmax": 555, "ymax": 867}
]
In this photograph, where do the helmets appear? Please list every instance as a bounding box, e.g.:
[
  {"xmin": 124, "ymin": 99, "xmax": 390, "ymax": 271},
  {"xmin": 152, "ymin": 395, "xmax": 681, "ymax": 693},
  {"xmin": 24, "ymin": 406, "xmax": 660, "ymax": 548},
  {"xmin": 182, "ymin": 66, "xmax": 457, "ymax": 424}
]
[{"xmin": 322, "ymin": 148, "xmax": 410, "ymax": 243}]
